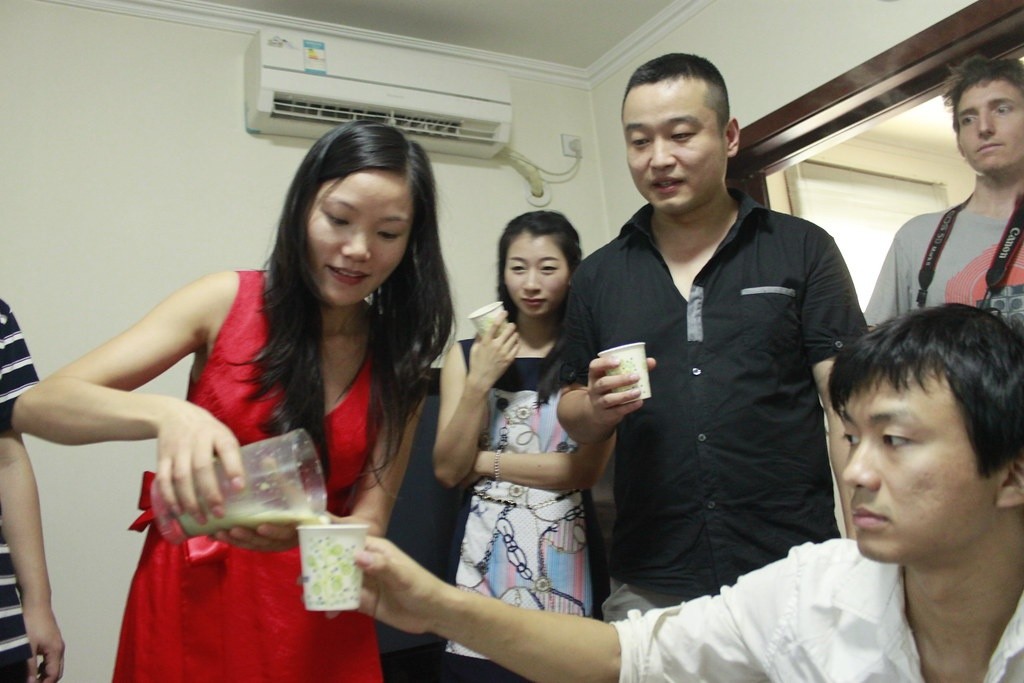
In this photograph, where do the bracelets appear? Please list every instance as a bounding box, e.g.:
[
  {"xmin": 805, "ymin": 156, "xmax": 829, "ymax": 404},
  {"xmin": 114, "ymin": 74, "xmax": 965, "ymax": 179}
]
[{"xmin": 493, "ymin": 449, "xmax": 503, "ymax": 481}]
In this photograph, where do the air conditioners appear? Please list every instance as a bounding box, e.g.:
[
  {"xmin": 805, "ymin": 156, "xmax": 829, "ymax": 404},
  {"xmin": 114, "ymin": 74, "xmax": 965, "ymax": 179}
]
[{"xmin": 243, "ymin": 27, "xmax": 510, "ymax": 160}]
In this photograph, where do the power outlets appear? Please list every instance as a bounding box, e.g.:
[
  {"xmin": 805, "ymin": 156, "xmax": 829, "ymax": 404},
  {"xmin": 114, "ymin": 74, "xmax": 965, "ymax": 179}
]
[{"xmin": 562, "ymin": 134, "xmax": 582, "ymax": 158}]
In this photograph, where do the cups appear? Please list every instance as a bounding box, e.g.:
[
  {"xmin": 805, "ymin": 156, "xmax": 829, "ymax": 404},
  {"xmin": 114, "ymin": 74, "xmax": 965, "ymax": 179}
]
[
  {"xmin": 150, "ymin": 428, "xmax": 327, "ymax": 542},
  {"xmin": 468, "ymin": 301, "xmax": 508, "ymax": 340},
  {"xmin": 597, "ymin": 342, "xmax": 652, "ymax": 402},
  {"xmin": 295, "ymin": 524, "xmax": 370, "ymax": 611}
]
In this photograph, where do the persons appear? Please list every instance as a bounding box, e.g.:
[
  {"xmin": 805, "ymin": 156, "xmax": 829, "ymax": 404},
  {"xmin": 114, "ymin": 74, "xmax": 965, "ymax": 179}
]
[{"xmin": 0, "ymin": 52, "xmax": 1024, "ymax": 683}]
